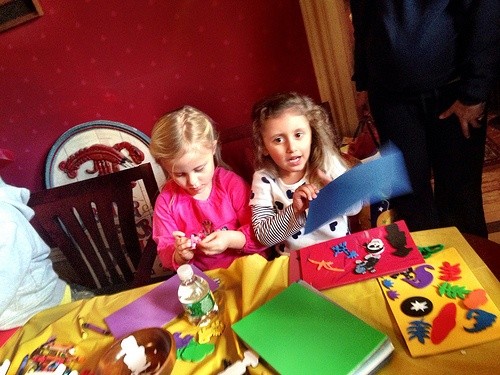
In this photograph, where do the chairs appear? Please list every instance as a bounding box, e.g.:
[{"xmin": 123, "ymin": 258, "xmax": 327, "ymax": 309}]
[
  {"xmin": 20, "ymin": 163, "xmax": 161, "ymax": 291},
  {"xmin": 42, "ymin": 119, "xmax": 174, "ymax": 282}
]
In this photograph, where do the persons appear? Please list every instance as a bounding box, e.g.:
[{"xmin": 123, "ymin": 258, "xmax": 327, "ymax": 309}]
[
  {"xmin": 351, "ymin": 0, "xmax": 500, "ymax": 239},
  {"xmin": 149, "ymin": 105, "xmax": 271, "ymax": 272},
  {"xmin": 0, "ymin": 176, "xmax": 67, "ymax": 330},
  {"xmin": 248, "ymin": 91, "xmax": 363, "ymax": 257}
]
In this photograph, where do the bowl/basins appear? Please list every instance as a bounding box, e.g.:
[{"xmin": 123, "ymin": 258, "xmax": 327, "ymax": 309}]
[{"xmin": 95, "ymin": 327, "xmax": 176, "ymax": 375}]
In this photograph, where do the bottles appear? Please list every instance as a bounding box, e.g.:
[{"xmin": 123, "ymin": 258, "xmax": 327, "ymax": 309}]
[{"xmin": 177, "ymin": 264, "xmax": 220, "ymax": 327}]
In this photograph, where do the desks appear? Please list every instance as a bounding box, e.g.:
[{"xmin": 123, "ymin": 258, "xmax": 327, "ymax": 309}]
[{"xmin": 0, "ymin": 224, "xmax": 500, "ymax": 375}]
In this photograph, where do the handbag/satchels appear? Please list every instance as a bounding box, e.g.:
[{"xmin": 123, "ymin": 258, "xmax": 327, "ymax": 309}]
[{"xmin": 347, "ymin": 114, "xmax": 380, "ymax": 161}]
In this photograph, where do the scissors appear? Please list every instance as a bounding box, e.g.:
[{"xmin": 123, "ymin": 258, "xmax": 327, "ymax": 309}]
[{"xmin": 418, "ymin": 244, "xmax": 442, "ymax": 258}]
